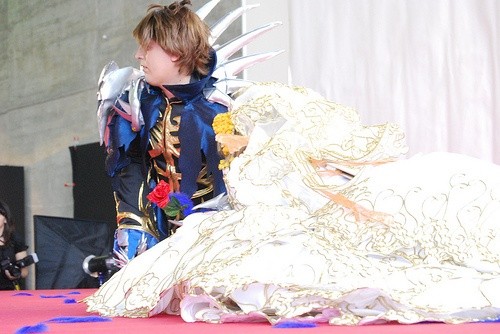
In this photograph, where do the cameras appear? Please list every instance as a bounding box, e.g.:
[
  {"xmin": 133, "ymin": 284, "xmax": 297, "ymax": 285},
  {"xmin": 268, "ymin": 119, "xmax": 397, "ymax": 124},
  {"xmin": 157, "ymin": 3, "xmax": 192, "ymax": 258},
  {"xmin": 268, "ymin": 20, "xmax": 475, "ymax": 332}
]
[{"xmin": 1, "ymin": 253, "xmax": 40, "ymax": 280}]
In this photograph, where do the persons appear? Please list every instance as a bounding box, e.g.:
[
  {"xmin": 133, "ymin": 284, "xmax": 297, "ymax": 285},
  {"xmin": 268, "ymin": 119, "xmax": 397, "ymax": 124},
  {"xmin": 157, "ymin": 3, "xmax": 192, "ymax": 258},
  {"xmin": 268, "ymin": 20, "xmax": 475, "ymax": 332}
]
[
  {"xmin": 0, "ymin": 200, "xmax": 29, "ymax": 293},
  {"xmin": 94, "ymin": 1, "xmax": 249, "ymax": 292}
]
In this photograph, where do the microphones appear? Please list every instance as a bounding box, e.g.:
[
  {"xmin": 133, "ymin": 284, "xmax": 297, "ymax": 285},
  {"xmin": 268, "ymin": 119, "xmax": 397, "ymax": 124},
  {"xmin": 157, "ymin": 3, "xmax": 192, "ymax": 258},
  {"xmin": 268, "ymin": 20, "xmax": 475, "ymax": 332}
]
[{"xmin": 82, "ymin": 254, "xmax": 115, "ymax": 278}]
